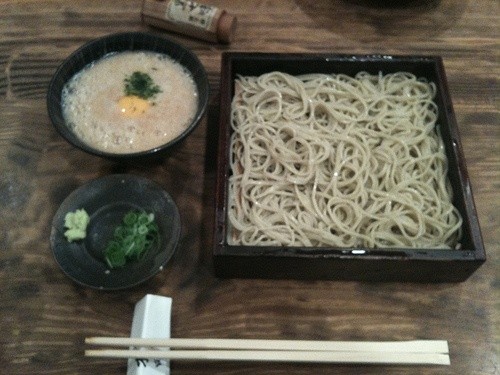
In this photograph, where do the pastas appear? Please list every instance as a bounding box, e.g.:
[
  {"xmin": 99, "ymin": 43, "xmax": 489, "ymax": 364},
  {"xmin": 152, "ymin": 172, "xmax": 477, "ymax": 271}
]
[{"xmin": 225, "ymin": 69, "xmax": 464, "ymax": 250}]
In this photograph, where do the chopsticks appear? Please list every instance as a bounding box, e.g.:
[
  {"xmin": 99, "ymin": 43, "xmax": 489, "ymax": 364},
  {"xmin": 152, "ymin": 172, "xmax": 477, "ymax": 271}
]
[{"xmin": 84, "ymin": 336, "xmax": 450, "ymax": 366}]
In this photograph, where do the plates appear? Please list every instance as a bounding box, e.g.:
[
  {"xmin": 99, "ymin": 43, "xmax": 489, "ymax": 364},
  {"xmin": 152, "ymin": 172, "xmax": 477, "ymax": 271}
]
[{"xmin": 49, "ymin": 174, "xmax": 183, "ymax": 291}]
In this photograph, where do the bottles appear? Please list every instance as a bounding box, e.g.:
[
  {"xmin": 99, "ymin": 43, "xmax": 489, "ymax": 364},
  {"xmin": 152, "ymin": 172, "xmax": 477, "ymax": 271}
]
[{"xmin": 142, "ymin": 0, "xmax": 237, "ymax": 44}]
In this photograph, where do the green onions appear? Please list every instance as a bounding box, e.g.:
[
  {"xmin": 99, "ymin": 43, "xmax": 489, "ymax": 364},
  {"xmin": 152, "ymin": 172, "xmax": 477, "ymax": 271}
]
[{"xmin": 104, "ymin": 210, "xmax": 159, "ymax": 269}]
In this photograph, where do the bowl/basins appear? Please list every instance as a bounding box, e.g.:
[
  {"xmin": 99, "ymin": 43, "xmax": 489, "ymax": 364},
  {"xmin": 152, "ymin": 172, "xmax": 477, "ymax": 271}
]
[{"xmin": 48, "ymin": 32, "xmax": 211, "ymax": 162}]
